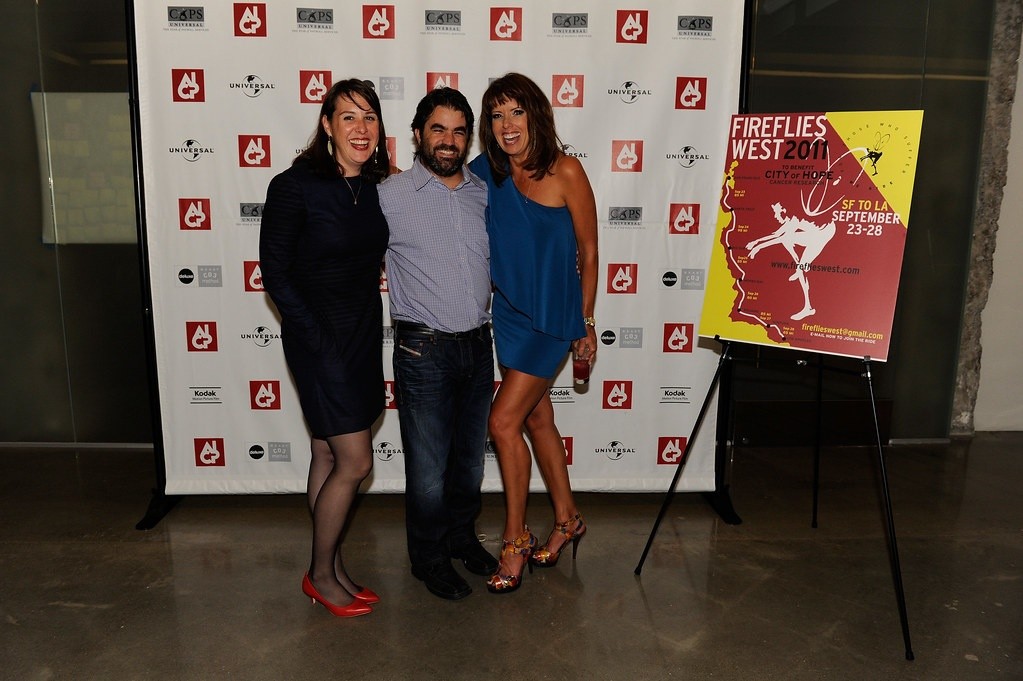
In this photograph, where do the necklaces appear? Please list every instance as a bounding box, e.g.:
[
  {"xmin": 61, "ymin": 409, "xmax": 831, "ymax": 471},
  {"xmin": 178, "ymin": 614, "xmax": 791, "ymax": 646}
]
[
  {"xmin": 512, "ymin": 170, "xmax": 533, "ymax": 204},
  {"xmin": 343, "ymin": 176, "xmax": 361, "ymax": 204}
]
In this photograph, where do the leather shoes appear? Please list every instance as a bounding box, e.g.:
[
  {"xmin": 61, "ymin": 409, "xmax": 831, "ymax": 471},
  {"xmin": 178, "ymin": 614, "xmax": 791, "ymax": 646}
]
[
  {"xmin": 411, "ymin": 560, "xmax": 472, "ymax": 600},
  {"xmin": 450, "ymin": 542, "xmax": 499, "ymax": 576}
]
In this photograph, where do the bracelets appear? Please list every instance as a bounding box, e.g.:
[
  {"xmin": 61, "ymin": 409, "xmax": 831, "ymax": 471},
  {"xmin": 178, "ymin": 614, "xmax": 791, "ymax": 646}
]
[{"xmin": 584, "ymin": 316, "xmax": 595, "ymax": 326}]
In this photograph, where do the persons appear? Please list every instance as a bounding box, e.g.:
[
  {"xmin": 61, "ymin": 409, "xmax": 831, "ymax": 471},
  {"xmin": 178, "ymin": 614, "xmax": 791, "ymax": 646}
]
[
  {"xmin": 376, "ymin": 86, "xmax": 496, "ymax": 602},
  {"xmin": 258, "ymin": 78, "xmax": 390, "ymax": 619},
  {"xmin": 466, "ymin": 72, "xmax": 599, "ymax": 594}
]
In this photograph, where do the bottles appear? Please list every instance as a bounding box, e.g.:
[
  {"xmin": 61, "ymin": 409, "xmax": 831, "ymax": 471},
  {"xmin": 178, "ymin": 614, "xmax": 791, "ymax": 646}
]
[{"xmin": 572, "ymin": 346, "xmax": 588, "ymax": 394}]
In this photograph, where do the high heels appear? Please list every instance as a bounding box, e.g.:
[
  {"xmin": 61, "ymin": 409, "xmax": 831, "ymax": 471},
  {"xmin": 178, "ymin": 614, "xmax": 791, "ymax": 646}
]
[
  {"xmin": 302, "ymin": 571, "xmax": 373, "ymax": 618},
  {"xmin": 487, "ymin": 524, "xmax": 538, "ymax": 593},
  {"xmin": 351, "ymin": 585, "xmax": 380, "ymax": 605},
  {"xmin": 532, "ymin": 512, "xmax": 587, "ymax": 567}
]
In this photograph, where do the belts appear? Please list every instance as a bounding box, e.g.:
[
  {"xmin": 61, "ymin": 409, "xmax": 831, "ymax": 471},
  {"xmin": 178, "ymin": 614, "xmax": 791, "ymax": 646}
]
[{"xmin": 393, "ymin": 320, "xmax": 488, "ymax": 341}]
